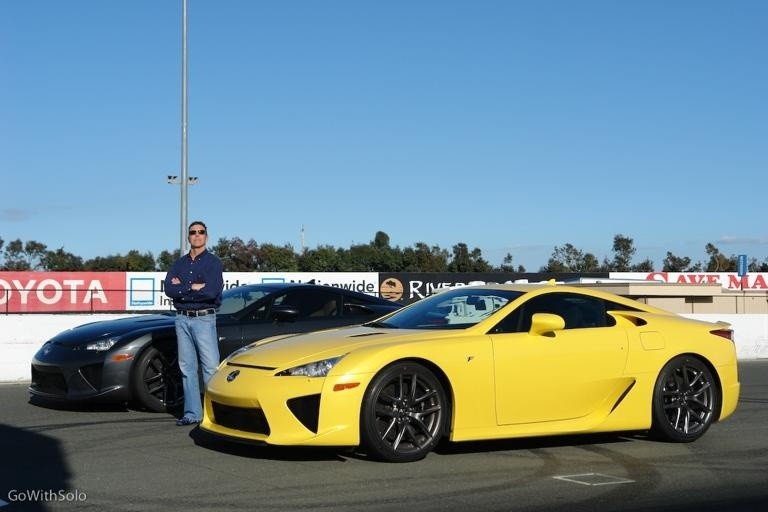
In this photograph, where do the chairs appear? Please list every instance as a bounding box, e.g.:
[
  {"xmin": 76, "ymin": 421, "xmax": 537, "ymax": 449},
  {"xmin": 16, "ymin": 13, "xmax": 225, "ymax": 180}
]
[{"xmin": 302, "ymin": 297, "xmax": 337, "ymax": 319}]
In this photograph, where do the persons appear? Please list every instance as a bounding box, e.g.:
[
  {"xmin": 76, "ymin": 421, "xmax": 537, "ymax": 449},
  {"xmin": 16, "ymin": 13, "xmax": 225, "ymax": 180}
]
[{"xmin": 164, "ymin": 221, "xmax": 225, "ymax": 427}]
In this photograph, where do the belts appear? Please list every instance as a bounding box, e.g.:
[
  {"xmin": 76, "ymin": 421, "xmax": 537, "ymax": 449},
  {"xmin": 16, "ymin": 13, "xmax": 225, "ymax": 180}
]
[{"xmin": 176, "ymin": 309, "xmax": 215, "ymax": 317}]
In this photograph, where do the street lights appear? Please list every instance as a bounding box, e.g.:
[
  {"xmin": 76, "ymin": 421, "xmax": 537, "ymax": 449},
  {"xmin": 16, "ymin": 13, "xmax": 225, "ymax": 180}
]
[{"xmin": 167, "ymin": 172, "xmax": 199, "ymax": 256}]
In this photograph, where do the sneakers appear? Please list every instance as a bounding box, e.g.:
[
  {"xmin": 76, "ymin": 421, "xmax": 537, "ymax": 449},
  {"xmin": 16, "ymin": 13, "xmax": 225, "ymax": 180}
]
[{"xmin": 177, "ymin": 417, "xmax": 202, "ymax": 425}]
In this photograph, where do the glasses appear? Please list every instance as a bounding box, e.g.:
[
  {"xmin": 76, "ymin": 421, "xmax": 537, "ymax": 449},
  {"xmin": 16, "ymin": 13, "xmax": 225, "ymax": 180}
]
[{"xmin": 189, "ymin": 230, "xmax": 206, "ymax": 235}]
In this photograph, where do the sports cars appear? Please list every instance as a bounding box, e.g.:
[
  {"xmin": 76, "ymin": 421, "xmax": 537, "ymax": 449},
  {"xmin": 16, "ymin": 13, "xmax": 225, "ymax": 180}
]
[
  {"xmin": 28, "ymin": 278, "xmax": 448, "ymax": 413},
  {"xmin": 199, "ymin": 278, "xmax": 740, "ymax": 463}
]
